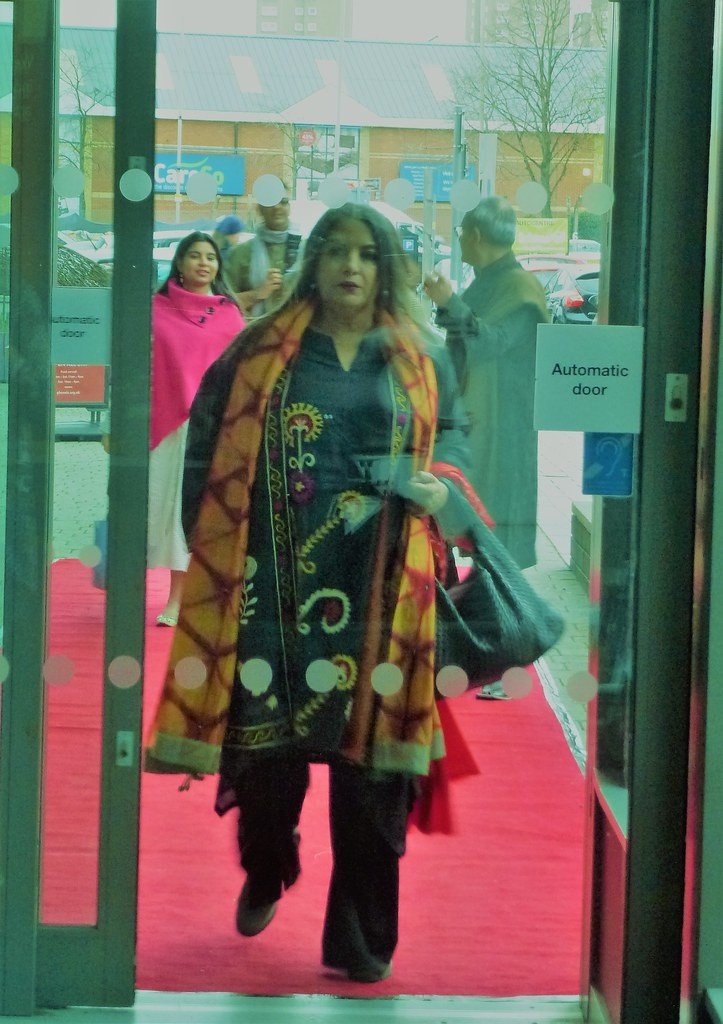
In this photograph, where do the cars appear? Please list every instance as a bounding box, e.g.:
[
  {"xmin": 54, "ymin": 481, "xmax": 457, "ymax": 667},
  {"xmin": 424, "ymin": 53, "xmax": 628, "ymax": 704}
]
[
  {"xmin": 57, "ymin": 227, "xmax": 258, "ymax": 293},
  {"xmin": 416, "ymin": 239, "xmax": 600, "ymax": 325}
]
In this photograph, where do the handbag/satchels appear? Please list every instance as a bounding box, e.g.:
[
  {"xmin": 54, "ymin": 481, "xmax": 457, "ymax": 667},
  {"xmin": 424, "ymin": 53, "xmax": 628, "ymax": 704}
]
[
  {"xmin": 92, "ymin": 520, "xmax": 106, "ymax": 592},
  {"xmin": 434, "ymin": 478, "xmax": 565, "ymax": 701}
]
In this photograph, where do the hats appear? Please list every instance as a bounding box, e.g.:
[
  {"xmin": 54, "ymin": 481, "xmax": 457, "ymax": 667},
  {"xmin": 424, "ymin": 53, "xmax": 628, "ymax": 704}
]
[{"xmin": 217, "ymin": 216, "xmax": 242, "ymax": 237}]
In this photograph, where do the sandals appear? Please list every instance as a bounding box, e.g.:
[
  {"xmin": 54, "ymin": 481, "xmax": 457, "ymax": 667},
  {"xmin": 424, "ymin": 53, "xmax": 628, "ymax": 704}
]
[{"xmin": 477, "ymin": 680, "xmax": 508, "ymax": 699}]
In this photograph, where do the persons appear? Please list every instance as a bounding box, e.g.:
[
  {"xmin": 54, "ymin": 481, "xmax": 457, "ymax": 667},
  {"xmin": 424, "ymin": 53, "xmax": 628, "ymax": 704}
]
[
  {"xmin": 150, "ymin": 178, "xmax": 548, "ymax": 696},
  {"xmin": 181, "ymin": 199, "xmax": 472, "ymax": 982}
]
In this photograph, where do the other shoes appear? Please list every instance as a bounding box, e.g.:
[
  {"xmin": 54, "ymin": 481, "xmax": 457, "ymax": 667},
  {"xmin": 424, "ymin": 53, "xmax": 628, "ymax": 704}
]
[
  {"xmin": 156, "ymin": 615, "xmax": 177, "ymax": 626},
  {"xmin": 236, "ymin": 870, "xmax": 275, "ymax": 938},
  {"xmin": 347, "ymin": 959, "xmax": 392, "ymax": 982}
]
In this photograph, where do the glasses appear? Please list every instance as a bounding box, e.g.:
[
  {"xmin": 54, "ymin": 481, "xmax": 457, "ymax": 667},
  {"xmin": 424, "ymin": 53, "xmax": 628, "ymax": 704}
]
[
  {"xmin": 454, "ymin": 226, "xmax": 468, "ymax": 238},
  {"xmin": 281, "ymin": 198, "xmax": 288, "ymax": 204}
]
